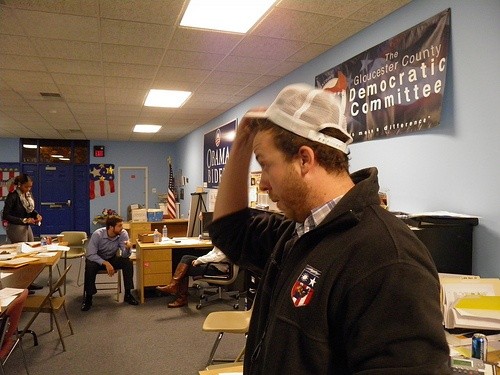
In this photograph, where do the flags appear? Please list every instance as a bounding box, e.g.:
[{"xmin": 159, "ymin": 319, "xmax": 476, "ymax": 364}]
[{"xmin": 166, "ymin": 166, "xmax": 177, "ymax": 218}]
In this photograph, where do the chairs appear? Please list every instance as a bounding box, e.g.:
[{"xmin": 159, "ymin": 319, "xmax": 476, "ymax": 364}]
[
  {"xmin": 0, "ymin": 289, "xmax": 30, "ymax": 375},
  {"xmin": 59, "ymin": 231, "xmax": 88, "ymax": 287},
  {"xmin": 16, "ymin": 262, "xmax": 74, "ymax": 354},
  {"xmin": 81, "ymin": 247, "xmax": 122, "ymax": 303},
  {"xmin": 193, "ymin": 261, "xmax": 240, "ymax": 310},
  {"xmin": 203, "ymin": 293, "xmax": 257, "ymax": 368}
]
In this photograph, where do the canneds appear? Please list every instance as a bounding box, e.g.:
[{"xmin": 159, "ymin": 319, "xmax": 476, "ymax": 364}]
[{"xmin": 471, "ymin": 333, "xmax": 488, "ymax": 363}]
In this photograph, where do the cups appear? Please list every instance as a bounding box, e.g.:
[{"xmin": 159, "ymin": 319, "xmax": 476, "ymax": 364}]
[
  {"xmin": 46, "ymin": 237, "xmax": 52, "ymax": 245},
  {"xmin": 57, "ymin": 234, "xmax": 65, "ymax": 244}
]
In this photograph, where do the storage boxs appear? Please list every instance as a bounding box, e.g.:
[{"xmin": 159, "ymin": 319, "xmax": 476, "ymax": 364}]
[
  {"xmin": 138, "ymin": 232, "xmax": 163, "ymax": 243},
  {"xmin": 147, "ymin": 208, "xmax": 164, "ymax": 222},
  {"xmin": 158, "ymin": 193, "xmax": 178, "ymax": 202},
  {"xmin": 131, "ymin": 204, "xmax": 138, "ymax": 210},
  {"xmin": 127, "ymin": 208, "xmax": 147, "ymax": 222}
]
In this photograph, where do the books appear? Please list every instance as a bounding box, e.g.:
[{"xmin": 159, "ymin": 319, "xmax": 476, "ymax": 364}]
[{"xmin": 438, "ymin": 273, "xmax": 500, "ymax": 330}]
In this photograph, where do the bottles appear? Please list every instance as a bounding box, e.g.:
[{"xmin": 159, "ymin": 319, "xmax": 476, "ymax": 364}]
[
  {"xmin": 41, "ymin": 235, "xmax": 47, "ymax": 253},
  {"xmin": 162, "ymin": 225, "xmax": 168, "ymax": 237},
  {"xmin": 154, "ymin": 229, "xmax": 159, "ymax": 244}
]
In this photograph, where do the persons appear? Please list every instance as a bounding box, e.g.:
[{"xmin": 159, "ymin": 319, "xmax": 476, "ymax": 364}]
[
  {"xmin": 81, "ymin": 215, "xmax": 139, "ymax": 311},
  {"xmin": 1, "ymin": 174, "xmax": 44, "ymax": 294},
  {"xmin": 156, "ymin": 246, "xmax": 230, "ymax": 308},
  {"xmin": 209, "ymin": 83, "xmax": 454, "ymax": 375}
]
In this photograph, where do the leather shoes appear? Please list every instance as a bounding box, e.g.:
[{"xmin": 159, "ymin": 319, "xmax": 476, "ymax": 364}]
[
  {"xmin": 124, "ymin": 294, "xmax": 138, "ymax": 305},
  {"xmin": 81, "ymin": 300, "xmax": 92, "ymax": 311}
]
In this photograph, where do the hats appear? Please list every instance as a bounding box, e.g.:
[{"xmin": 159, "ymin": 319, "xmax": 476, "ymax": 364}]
[{"xmin": 244, "ymin": 83, "xmax": 353, "ymax": 155}]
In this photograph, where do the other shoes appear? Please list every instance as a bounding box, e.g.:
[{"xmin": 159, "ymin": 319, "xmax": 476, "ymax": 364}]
[{"xmin": 28, "ymin": 282, "xmax": 43, "ymax": 290}]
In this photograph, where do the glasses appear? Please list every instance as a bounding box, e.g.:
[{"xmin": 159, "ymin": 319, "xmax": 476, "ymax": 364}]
[{"xmin": 22, "ymin": 184, "xmax": 33, "ymax": 188}]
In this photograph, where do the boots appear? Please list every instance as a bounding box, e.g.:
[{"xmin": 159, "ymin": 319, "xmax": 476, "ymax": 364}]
[
  {"xmin": 156, "ymin": 263, "xmax": 188, "ymax": 296},
  {"xmin": 168, "ymin": 281, "xmax": 189, "ymax": 308}
]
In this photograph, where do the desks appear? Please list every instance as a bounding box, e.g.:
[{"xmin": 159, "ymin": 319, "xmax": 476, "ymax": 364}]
[
  {"xmin": 0, "ymin": 263, "xmax": 46, "ymax": 346},
  {"xmin": 135, "ymin": 236, "xmax": 214, "ymax": 306},
  {"xmin": 0, "ymin": 241, "xmax": 69, "ymax": 332},
  {"xmin": 154, "ymin": 202, "xmax": 180, "ymax": 219},
  {"xmin": 129, "ymin": 218, "xmax": 188, "ymax": 243}
]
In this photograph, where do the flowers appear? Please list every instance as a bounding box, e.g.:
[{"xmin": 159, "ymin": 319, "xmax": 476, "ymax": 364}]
[{"xmin": 92, "ymin": 208, "xmax": 119, "ymax": 224}]
